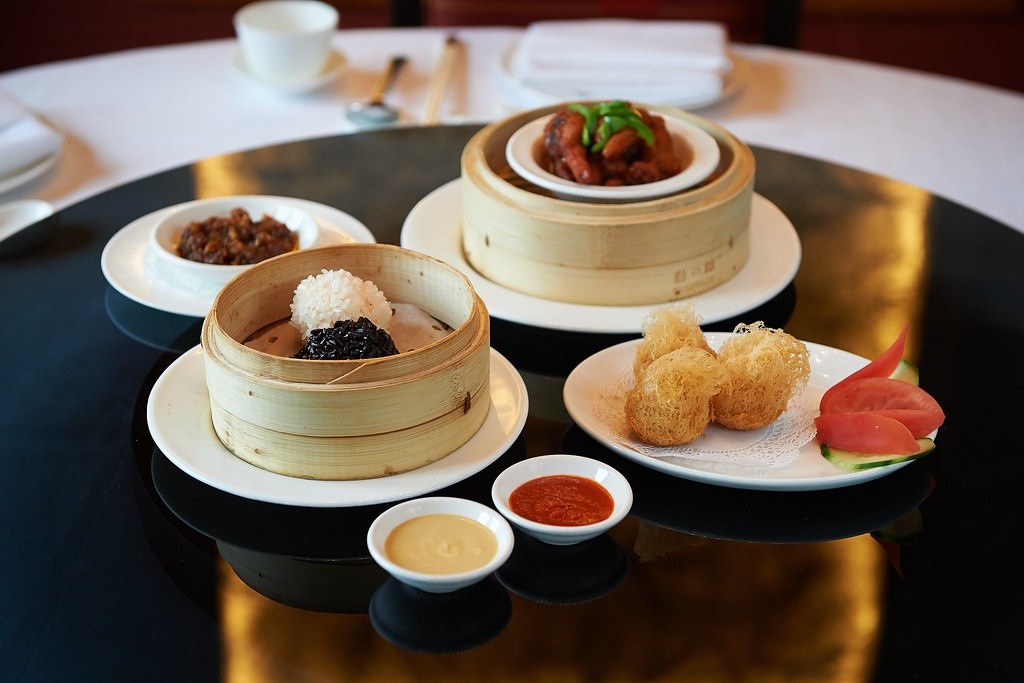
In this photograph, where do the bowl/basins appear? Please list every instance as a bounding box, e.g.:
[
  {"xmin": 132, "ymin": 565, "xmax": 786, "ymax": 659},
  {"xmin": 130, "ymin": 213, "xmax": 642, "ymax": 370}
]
[{"xmin": 150, "ymin": 197, "xmax": 320, "ymax": 292}]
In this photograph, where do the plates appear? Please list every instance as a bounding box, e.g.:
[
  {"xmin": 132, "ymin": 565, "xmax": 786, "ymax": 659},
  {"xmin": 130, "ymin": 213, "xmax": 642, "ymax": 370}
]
[
  {"xmin": 506, "ymin": 113, "xmax": 720, "ymax": 202},
  {"xmin": 563, "ymin": 334, "xmax": 938, "ymax": 490},
  {"xmin": 510, "ymin": 21, "xmax": 728, "ymax": 110},
  {"xmin": 229, "ymin": 45, "xmax": 352, "ymax": 91},
  {"xmin": 101, "ymin": 198, "xmax": 376, "ymax": 318},
  {"xmin": 367, "ymin": 496, "xmax": 514, "ymax": 593},
  {"xmin": 0, "ymin": 104, "xmax": 65, "ymax": 194},
  {"xmin": 400, "ymin": 177, "xmax": 803, "ymax": 333},
  {"xmin": 147, "ymin": 344, "xmax": 529, "ymax": 508},
  {"xmin": 491, "ymin": 455, "xmax": 634, "ymax": 546}
]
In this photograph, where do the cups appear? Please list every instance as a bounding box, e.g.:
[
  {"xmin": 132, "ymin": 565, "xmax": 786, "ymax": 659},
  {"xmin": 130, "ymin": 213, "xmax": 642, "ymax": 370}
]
[{"xmin": 233, "ymin": 0, "xmax": 339, "ymax": 83}]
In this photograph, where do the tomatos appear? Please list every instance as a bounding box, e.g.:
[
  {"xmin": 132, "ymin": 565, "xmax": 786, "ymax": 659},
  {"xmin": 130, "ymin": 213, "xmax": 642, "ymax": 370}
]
[{"xmin": 815, "ymin": 327, "xmax": 945, "ymax": 455}]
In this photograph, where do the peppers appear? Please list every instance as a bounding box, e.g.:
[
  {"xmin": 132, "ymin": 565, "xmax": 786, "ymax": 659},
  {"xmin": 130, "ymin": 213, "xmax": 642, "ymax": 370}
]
[{"xmin": 568, "ymin": 99, "xmax": 654, "ymax": 151}]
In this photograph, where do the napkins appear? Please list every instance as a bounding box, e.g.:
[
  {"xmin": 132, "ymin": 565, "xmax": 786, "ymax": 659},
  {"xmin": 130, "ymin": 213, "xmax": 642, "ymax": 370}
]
[
  {"xmin": 0, "ymin": 89, "xmax": 62, "ymax": 180},
  {"xmin": 515, "ymin": 17, "xmax": 727, "ymax": 105}
]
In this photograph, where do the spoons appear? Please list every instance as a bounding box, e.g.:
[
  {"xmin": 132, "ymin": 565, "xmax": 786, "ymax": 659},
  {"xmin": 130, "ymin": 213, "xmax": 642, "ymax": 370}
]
[{"xmin": 345, "ymin": 56, "xmax": 408, "ymax": 126}]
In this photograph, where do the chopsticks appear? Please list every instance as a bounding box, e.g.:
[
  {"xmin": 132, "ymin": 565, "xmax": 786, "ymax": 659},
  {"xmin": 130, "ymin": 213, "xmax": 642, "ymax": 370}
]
[{"xmin": 422, "ymin": 38, "xmax": 467, "ymax": 127}]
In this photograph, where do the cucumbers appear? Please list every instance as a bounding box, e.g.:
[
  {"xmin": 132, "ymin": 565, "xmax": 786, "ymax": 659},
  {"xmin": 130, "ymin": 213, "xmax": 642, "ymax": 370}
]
[
  {"xmin": 821, "ymin": 437, "xmax": 936, "ymax": 470},
  {"xmin": 889, "ymin": 361, "xmax": 920, "ymax": 385}
]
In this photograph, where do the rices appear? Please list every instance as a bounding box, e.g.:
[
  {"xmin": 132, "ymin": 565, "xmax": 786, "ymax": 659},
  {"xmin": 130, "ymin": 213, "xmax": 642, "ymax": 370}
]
[{"xmin": 288, "ymin": 268, "xmax": 399, "ymax": 359}]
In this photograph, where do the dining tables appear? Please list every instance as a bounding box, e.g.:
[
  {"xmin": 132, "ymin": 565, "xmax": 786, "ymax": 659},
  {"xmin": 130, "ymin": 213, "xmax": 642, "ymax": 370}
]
[{"xmin": 1, "ymin": 28, "xmax": 1024, "ymax": 683}]
